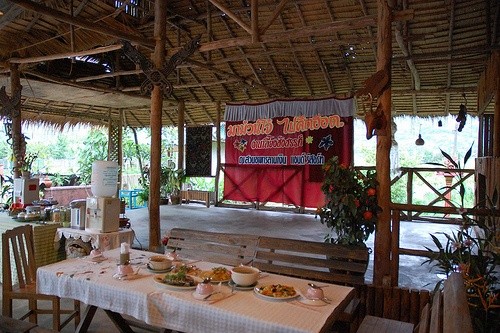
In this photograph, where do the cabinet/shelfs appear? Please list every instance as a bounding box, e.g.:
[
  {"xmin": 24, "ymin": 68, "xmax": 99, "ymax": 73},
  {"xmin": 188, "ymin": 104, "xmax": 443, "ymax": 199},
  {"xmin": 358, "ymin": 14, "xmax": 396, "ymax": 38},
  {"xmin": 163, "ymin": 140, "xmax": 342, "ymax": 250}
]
[{"xmin": 118, "ymin": 188, "xmax": 147, "ymax": 210}]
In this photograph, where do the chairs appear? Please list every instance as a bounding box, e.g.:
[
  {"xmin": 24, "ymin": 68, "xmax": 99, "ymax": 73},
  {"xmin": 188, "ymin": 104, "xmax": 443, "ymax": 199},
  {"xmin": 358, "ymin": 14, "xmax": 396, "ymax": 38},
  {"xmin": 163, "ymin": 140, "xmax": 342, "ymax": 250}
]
[
  {"xmin": 2, "ymin": 223, "xmax": 81, "ymax": 333},
  {"xmin": 32, "ymin": 224, "xmax": 63, "ymax": 267}
]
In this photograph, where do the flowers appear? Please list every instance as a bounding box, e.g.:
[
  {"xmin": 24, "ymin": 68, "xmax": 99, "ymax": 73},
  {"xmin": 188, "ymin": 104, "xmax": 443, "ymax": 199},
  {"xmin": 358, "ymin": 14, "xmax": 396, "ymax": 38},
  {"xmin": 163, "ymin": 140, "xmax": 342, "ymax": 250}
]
[{"xmin": 313, "ymin": 153, "xmax": 383, "ymax": 245}]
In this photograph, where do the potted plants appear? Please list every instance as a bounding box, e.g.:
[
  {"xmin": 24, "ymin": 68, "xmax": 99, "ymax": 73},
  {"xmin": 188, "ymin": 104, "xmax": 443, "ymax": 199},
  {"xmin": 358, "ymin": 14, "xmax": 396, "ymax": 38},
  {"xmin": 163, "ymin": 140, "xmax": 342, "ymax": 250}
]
[
  {"xmin": 9, "ymin": 149, "xmax": 39, "ymax": 178},
  {"xmin": 137, "ymin": 163, "xmax": 187, "ymax": 206}
]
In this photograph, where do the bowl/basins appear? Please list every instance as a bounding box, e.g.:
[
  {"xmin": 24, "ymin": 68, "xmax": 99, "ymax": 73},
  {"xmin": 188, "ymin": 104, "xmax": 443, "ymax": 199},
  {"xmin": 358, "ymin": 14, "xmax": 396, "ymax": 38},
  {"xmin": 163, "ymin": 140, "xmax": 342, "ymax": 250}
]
[
  {"xmin": 148, "ymin": 255, "xmax": 174, "ymax": 270},
  {"xmin": 231, "ymin": 267, "xmax": 260, "ymax": 286}
]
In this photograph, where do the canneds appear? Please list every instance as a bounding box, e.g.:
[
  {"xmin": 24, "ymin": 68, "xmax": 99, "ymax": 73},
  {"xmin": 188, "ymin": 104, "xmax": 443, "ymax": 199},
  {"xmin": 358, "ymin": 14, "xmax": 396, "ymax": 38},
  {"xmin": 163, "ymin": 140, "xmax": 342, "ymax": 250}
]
[{"xmin": 54, "ymin": 212, "xmax": 65, "ymax": 222}]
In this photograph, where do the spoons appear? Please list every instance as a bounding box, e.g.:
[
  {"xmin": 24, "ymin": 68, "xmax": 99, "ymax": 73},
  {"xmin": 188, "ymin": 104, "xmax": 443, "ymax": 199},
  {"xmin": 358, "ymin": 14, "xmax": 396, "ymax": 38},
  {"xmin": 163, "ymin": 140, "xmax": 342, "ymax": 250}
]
[
  {"xmin": 307, "ymin": 283, "xmax": 329, "ymax": 289},
  {"xmin": 199, "ymin": 277, "xmax": 212, "ymax": 283}
]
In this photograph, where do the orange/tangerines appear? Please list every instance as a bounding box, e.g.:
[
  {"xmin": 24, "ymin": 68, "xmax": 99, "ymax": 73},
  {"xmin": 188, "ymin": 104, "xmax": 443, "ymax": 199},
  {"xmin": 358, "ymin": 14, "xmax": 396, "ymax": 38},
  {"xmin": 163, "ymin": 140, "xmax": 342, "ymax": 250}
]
[{"xmin": 316, "ymin": 164, "xmax": 375, "ymax": 220}]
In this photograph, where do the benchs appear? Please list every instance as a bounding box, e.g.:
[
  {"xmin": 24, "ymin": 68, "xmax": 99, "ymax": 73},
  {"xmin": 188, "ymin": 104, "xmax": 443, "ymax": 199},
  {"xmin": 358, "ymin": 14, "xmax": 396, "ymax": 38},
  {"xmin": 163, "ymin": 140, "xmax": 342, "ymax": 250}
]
[
  {"xmin": 252, "ymin": 237, "xmax": 371, "ymax": 333},
  {"xmin": 356, "ymin": 271, "xmax": 473, "ymax": 333},
  {"xmin": 165, "ymin": 226, "xmax": 258, "ymax": 268}
]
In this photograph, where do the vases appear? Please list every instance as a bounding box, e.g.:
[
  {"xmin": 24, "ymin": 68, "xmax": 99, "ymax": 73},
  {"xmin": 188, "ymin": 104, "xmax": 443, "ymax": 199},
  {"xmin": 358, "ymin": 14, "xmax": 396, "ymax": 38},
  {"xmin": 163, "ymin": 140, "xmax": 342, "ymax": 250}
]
[{"xmin": 325, "ymin": 242, "xmax": 370, "ymax": 280}]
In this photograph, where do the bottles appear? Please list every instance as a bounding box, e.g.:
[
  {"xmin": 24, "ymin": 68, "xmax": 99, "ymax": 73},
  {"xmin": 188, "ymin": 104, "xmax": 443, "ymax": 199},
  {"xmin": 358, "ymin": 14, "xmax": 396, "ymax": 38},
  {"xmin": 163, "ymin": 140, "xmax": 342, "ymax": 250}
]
[
  {"xmin": 44, "ymin": 205, "xmax": 71, "ymax": 222},
  {"xmin": 91, "ymin": 161, "xmax": 118, "ymax": 198}
]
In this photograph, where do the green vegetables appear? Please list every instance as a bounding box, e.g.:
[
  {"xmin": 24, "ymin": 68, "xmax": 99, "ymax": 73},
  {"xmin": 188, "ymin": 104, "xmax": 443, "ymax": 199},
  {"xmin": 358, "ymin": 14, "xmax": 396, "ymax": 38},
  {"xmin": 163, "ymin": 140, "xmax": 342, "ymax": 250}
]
[
  {"xmin": 212, "ymin": 267, "xmax": 226, "ymax": 274},
  {"xmin": 165, "ymin": 264, "xmax": 190, "ymax": 283},
  {"xmin": 259, "ymin": 284, "xmax": 288, "ymax": 292}
]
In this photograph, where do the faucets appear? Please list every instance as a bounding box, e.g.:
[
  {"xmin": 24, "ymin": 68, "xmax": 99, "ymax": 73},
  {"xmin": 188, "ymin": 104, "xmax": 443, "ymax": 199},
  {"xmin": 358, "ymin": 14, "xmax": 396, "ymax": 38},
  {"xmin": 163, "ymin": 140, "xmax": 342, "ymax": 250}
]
[
  {"xmin": 94, "ymin": 210, "xmax": 97, "ymax": 218},
  {"xmin": 86, "ymin": 208, "xmax": 90, "ymax": 217}
]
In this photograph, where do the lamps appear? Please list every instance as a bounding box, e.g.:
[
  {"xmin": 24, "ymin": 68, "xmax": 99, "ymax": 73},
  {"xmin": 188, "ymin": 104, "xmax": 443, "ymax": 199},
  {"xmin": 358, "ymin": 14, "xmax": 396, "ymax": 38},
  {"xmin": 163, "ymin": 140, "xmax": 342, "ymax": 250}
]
[{"xmin": 415, "ymin": 124, "xmax": 424, "ymax": 146}]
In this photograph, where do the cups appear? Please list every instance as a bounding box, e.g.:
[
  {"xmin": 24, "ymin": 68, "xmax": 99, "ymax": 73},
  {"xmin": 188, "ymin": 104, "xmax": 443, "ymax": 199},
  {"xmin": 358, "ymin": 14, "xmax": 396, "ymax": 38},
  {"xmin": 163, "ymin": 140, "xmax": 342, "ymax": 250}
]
[
  {"xmin": 196, "ymin": 283, "xmax": 214, "ymax": 296},
  {"xmin": 307, "ymin": 288, "xmax": 324, "ymax": 300}
]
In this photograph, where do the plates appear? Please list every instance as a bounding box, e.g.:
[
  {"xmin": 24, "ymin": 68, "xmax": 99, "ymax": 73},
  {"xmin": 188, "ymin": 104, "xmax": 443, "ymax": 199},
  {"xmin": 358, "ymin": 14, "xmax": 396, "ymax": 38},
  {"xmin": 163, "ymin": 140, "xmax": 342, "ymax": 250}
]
[
  {"xmin": 228, "ymin": 279, "xmax": 260, "ymax": 290},
  {"xmin": 298, "ymin": 295, "xmax": 328, "ymax": 306},
  {"xmin": 152, "ymin": 273, "xmax": 204, "ymax": 290},
  {"xmin": 200, "ymin": 270, "xmax": 230, "ymax": 284},
  {"xmin": 254, "ymin": 283, "xmax": 300, "ymax": 300},
  {"xmin": 191, "ymin": 291, "xmax": 224, "ymax": 300},
  {"xmin": 146, "ymin": 264, "xmax": 175, "ymax": 273}
]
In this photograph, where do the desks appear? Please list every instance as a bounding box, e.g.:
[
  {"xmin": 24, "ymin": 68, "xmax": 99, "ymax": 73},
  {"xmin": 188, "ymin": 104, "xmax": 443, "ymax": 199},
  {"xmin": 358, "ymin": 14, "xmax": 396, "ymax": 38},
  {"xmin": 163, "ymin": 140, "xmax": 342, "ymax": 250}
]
[
  {"xmin": 43, "ymin": 184, "xmax": 93, "ymax": 207},
  {"xmin": 34, "ymin": 247, "xmax": 356, "ymax": 333},
  {"xmin": 58, "ymin": 227, "xmax": 134, "ymax": 251}
]
